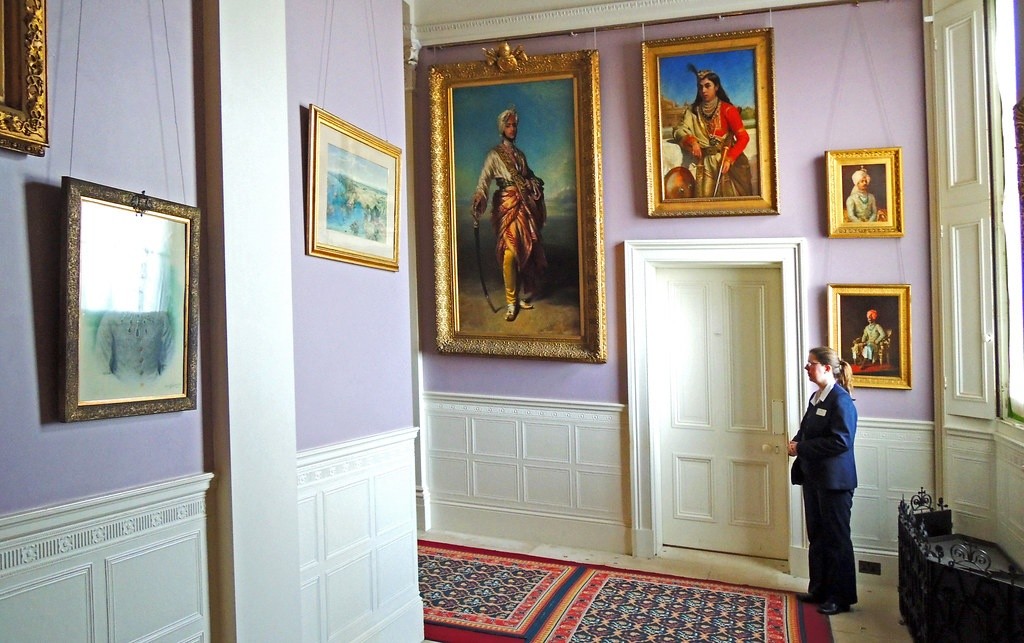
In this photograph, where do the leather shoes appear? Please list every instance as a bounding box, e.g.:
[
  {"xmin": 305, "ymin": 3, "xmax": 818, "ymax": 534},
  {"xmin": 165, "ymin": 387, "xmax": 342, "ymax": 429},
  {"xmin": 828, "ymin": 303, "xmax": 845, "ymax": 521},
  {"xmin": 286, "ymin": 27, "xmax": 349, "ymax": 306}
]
[
  {"xmin": 817, "ymin": 601, "xmax": 850, "ymax": 614},
  {"xmin": 796, "ymin": 592, "xmax": 827, "ymax": 604}
]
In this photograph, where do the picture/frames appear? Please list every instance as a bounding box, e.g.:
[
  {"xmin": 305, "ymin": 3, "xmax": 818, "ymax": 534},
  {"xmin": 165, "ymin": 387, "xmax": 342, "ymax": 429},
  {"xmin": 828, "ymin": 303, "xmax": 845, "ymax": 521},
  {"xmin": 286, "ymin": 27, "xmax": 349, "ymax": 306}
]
[
  {"xmin": 428, "ymin": 48, "xmax": 609, "ymax": 363},
  {"xmin": 305, "ymin": 104, "xmax": 402, "ymax": 272},
  {"xmin": 60, "ymin": 175, "xmax": 200, "ymax": 420},
  {"xmin": 825, "ymin": 147, "xmax": 904, "ymax": 239},
  {"xmin": 640, "ymin": 26, "xmax": 780, "ymax": 216},
  {"xmin": 827, "ymin": 283, "xmax": 914, "ymax": 391},
  {"xmin": 0, "ymin": 0, "xmax": 51, "ymax": 157}
]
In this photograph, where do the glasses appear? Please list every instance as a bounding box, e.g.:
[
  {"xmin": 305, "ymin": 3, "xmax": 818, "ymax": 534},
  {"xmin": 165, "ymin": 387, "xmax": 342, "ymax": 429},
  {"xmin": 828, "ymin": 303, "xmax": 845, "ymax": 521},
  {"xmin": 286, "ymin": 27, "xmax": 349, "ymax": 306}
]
[{"xmin": 808, "ymin": 361, "xmax": 818, "ymax": 366}]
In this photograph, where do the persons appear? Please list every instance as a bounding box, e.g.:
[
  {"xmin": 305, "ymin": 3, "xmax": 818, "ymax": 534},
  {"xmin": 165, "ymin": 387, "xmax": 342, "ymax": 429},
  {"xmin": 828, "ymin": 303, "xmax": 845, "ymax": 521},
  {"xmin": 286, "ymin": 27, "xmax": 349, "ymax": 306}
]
[{"xmin": 787, "ymin": 347, "xmax": 860, "ymax": 615}]
